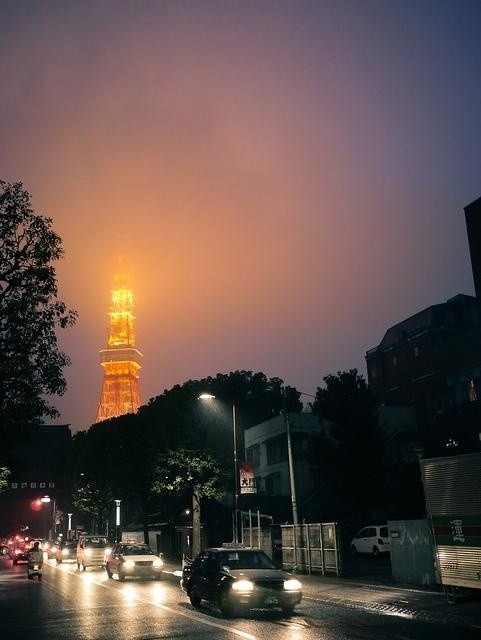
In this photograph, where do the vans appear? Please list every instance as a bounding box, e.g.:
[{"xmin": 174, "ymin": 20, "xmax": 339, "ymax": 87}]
[{"xmin": 76, "ymin": 535, "xmax": 109, "ymax": 571}]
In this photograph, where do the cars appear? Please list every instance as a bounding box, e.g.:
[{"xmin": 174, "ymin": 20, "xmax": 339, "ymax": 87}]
[
  {"xmin": 106, "ymin": 543, "xmax": 165, "ymax": 582},
  {"xmin": 350, "ymin": 525, "xmax": 390, "ymax": 559},
  {"xmin": 0, "ymin": 539, "xmax": 77, "ymax": 566},
  {"xmin": 187, "ymin": 548, "xmax": 303, "ymax": 619}
]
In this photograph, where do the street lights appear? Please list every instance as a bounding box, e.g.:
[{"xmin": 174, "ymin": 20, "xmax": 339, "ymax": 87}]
[
  {"xmin": 112, "ymin": 499, "xmax": 122, "ymax": 542},
  {"xmin": 196, "ymin": 390, "xmax": 240, "ymax": 511},
  {"xmin": 66, "ymin": 514, "xmax": 73, "ymax": 540},
  {"xmin": 41, "ymin": 495, "xmax": 56, "ymax": 537}
]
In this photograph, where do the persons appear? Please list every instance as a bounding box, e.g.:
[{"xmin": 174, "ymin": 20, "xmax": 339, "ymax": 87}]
[{"xmin": 29, "ymin": 540, "xmax": 43, "ymax": 569}]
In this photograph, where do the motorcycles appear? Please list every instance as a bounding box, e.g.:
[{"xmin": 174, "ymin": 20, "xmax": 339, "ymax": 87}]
[{"xmin": 26, "ymin": 550, "xmax": 44, "ymax": 580}]
